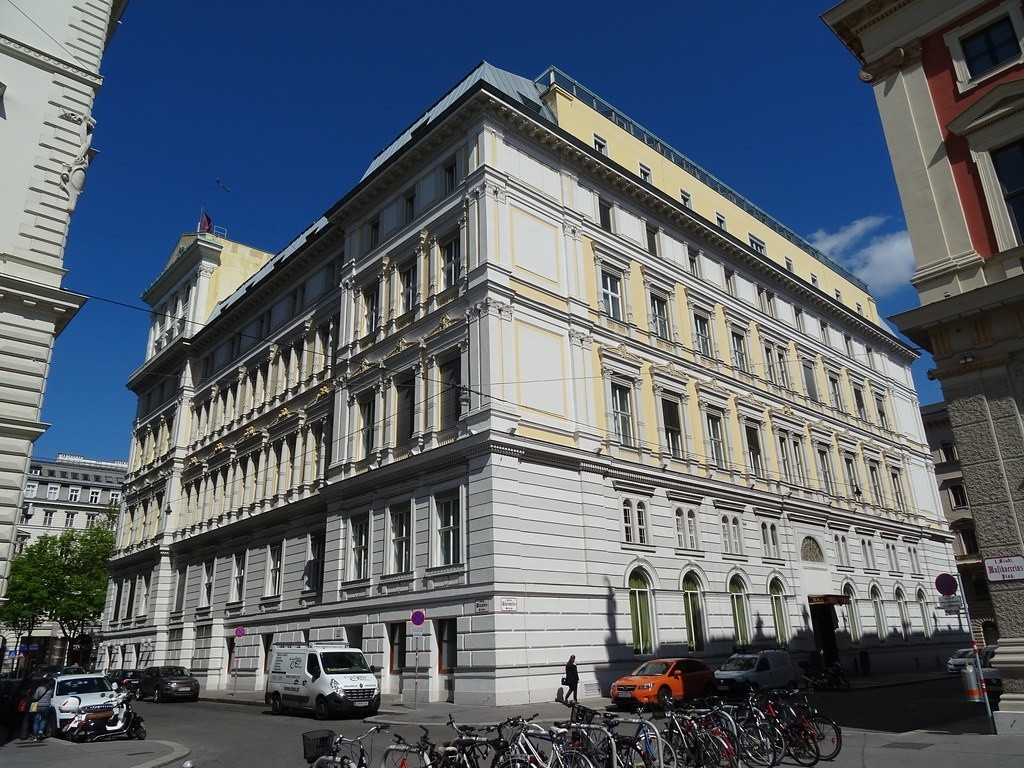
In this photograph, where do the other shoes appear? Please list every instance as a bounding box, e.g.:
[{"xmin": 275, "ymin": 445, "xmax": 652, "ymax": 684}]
[
  {"xmin": 34, "ymin": 737, "xmax": 38, "ymax": 742},
  {"xmin": 37, "ymin": 733, "xmax": 41, "ymax": 742}
]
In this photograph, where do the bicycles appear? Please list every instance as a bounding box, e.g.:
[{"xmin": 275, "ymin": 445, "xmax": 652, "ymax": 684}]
[{"xmin": 301, "ymin": 681, "xmax": 843, "ymax": 768}]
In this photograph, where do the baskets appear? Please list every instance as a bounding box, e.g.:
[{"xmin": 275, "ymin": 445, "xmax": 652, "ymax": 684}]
[
  {"xmin": 303, "ymin": 730, "xmax": 335, "ymax": 760},
  {"xmin": 570, "ymin": 704, "xmax": 595, "ymax": 724}
]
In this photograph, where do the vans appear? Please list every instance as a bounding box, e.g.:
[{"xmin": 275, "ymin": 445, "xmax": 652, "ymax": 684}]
[{"xmin": 263, "ymin": 637, "xmax": 381, "ymax": 721}]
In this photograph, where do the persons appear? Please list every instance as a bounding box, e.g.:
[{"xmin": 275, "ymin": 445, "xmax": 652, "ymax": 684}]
[
  {"xmin": 565, "ymin": 655, "xmax": 579, "ymax": 704},
  {"xmin": 6, "ymin": 677, "xmax": 35, "ymax": 740},
  {"xmin": 33, "ymin": 679, "xmax": 55, "ymax": 740}
]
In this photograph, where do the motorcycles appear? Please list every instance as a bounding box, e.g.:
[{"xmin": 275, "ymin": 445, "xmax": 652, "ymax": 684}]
[
  {"xmin": 58, "ymin": 678, "xmax": 148, "ymax": 742},
  {"xmin": 797, "ymin": 654, "xmax": 850, "ymax": 691}
]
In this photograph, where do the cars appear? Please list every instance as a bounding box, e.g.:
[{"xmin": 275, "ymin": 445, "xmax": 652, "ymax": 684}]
[
  {"xmin": 0, "ymin": 660, "xmax": 200, "ymax": 736},
  {"xmin": 608, "ymin": 656, "xmax": 719, "ymax": 711},
  {"xmin": 713, "ymin": 648, "xmax": 796, "ymax": 697},
  {"xmin": 946, "ymin": 644, "xmax": 1002, "ymax": 697}
]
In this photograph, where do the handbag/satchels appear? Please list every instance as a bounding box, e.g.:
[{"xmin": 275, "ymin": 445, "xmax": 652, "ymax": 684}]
[
  {"xmin": 28, "ymin": 702, "xmax": 38, "ymax": 713},
  {"xmin": 562, "ymin": 677, "xmax": 569, "ymax": 686},
  {"xmin": 18, "ymin": 697, "xmax": 28, "ymax": 712}
]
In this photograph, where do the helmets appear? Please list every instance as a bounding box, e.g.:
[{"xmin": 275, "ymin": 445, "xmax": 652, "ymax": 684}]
[{"xmin": 40, "ymin": 673, "xmax": 53, "ymax": 681}]
